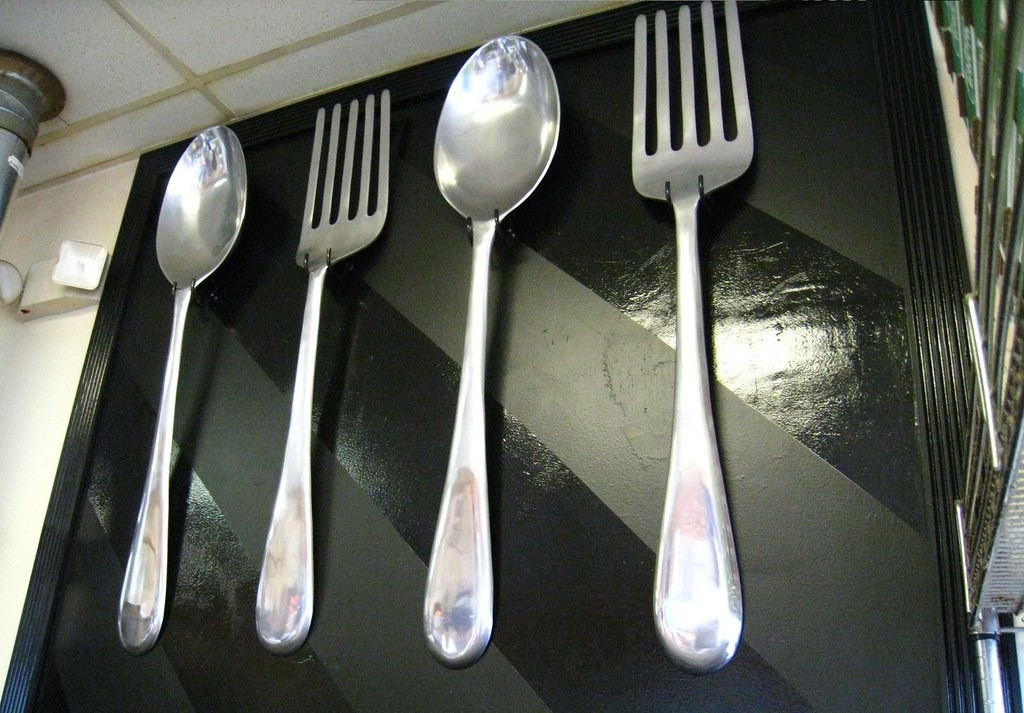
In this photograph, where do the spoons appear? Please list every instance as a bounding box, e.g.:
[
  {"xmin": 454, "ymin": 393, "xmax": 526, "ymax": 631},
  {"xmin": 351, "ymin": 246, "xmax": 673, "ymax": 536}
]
[
  {"xmin": 116, "ymin": 121, "xmax": 247, "ymax": 653},
  {"xmin": 424, "ymin": 35, "xmax": 560, "ymax": 662}
]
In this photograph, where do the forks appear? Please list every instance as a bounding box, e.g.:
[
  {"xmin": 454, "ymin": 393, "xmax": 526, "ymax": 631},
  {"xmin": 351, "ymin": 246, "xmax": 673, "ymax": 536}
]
[
  {"xmin": 256, "ymin": 89, "xmax": 392, "ymax": 648},
  {"xmin": 630, "ymin": 0, "xmax": 756, "ymax": 668}
]
